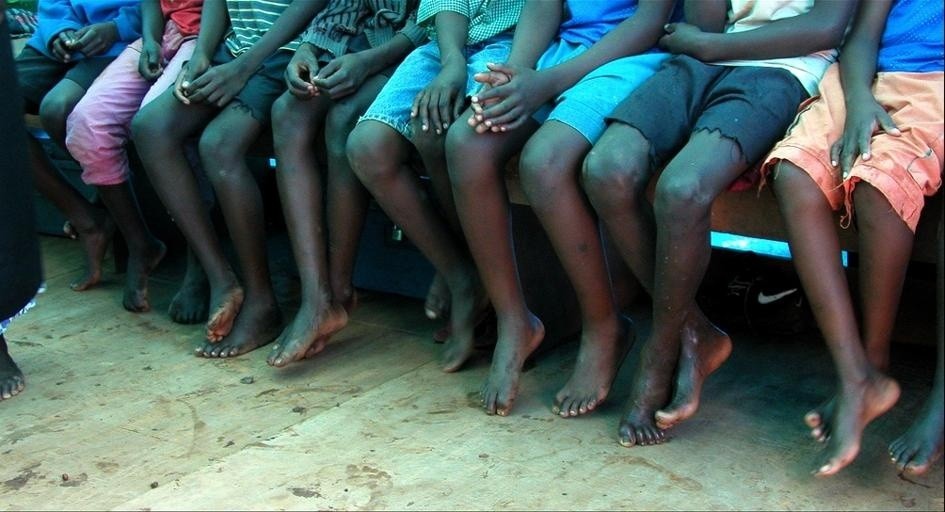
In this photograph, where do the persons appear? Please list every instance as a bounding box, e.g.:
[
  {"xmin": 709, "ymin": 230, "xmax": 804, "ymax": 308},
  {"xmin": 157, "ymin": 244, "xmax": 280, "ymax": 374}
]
[
  {"xmin": 347, "ymin": 0, "xmax": 526, "ymax": 373},
  {"xmin": 130, "ymin": 0, "xmax": 331, "ymax": 358},
  {"xmin": 582, "ymin": 0, "xmax": 855, "ymax": 448},
  {"xmin": 265, "ymin": 0, "xmax": 431, "ymax": 367},
  {"xmin": 760, "ymin": 0, "xmax": 945, "ymax": 476},
  {"xmin": 65, "ymin": 0, "xmax": 205, "ymax": 324},
  {"xmin": 888, "ymin": 210, "xmax": 944, "ymax": 475},
  {"xmin": 0, "ymin": 0, "xmax": 47, "ymax": 401},
  {"xmin": 13, "ymin": 0, "xmax": 142, "ymax": 291},
  {"xmin": 446, "ymin": 0, "xmax": 684, "ymax": 418}
]
